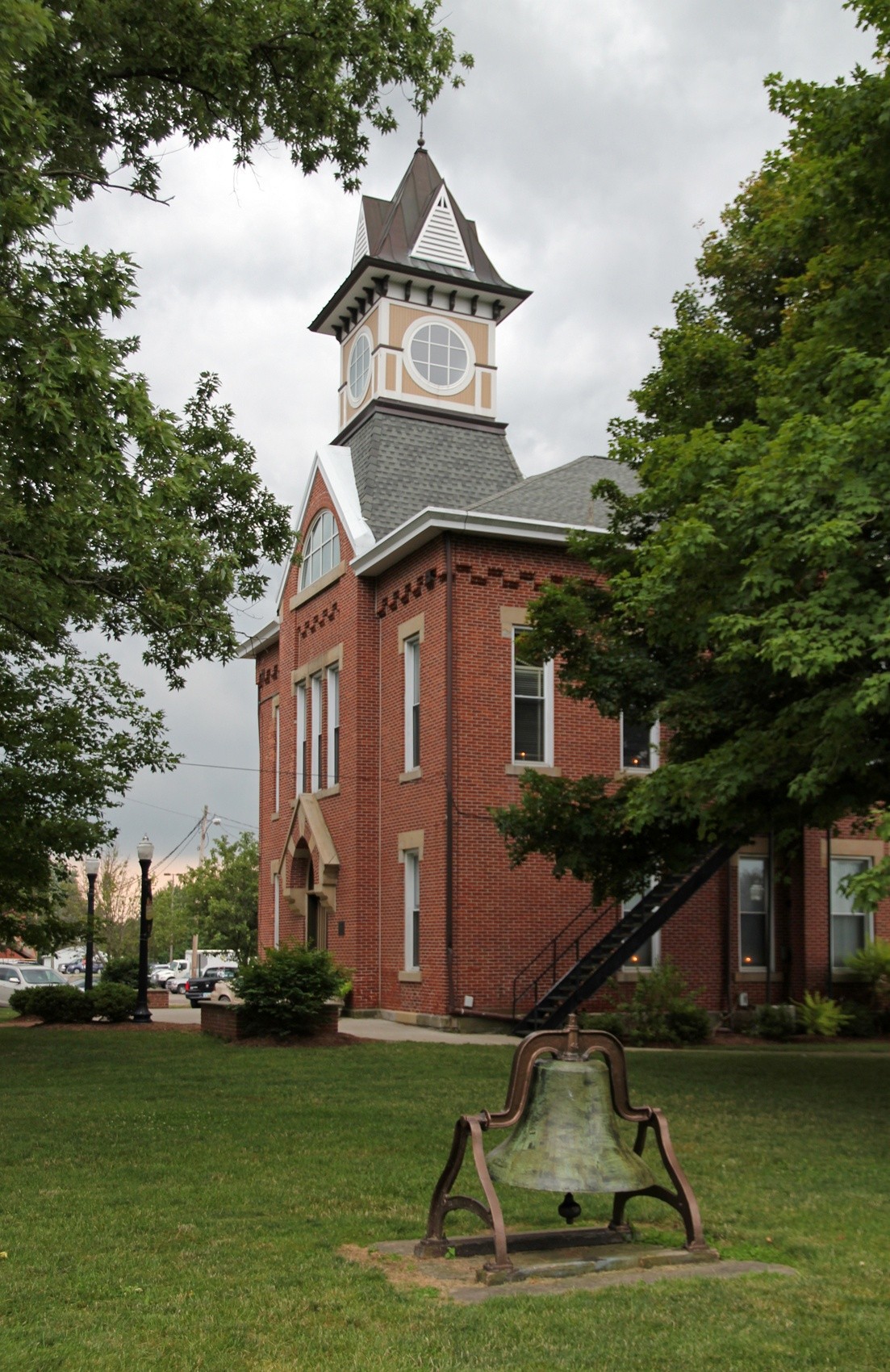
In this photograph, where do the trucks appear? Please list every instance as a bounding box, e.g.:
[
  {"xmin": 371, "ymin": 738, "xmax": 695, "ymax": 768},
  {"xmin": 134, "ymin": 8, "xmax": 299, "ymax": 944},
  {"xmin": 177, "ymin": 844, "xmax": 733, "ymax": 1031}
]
[{"xmin": 155, "ymin": 950, "xmax": 238, "ymax": 988}]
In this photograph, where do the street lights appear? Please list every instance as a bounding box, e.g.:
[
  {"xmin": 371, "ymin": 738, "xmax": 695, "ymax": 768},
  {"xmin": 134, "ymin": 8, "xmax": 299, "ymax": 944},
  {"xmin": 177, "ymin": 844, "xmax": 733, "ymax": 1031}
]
[
  {"xmin": 191, "ymin": 817, "xmax": 220, "ymax": 979},
  {"xmin": 131, "ymin": 832, "xmax": 153, "ymax": 1022},
  {"xmin": 164, "ymin": 873, "xmax": 183, "ymax": 961},
  {"xmin": 85, "ymin": 856, "xmax": 99, "ymax": 992}
]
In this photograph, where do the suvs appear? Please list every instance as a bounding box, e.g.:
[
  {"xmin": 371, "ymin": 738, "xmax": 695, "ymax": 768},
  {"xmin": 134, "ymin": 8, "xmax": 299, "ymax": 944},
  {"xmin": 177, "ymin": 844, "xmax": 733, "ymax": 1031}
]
[
  {"xmin": 185, "ymin": 964, "xmax": 238, "ymax": 1008},
  {"xmin": 148, "ymin": 962, "xmax": 170, "ymax": 987},
  {"xmin": 166, "ymin": 967, "xmax": 206, "ymax": 995}
]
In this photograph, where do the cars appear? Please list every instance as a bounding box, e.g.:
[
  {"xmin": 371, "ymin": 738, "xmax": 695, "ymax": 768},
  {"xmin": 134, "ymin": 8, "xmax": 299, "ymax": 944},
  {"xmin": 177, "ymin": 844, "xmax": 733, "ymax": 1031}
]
[
  {"xmin": 66, "ymin": 976, "xmax": 98, "ymax": 993},
  {"xmin": 58, "ymin": 957, "xmax": 104, "ymax": 974},
  {"xmin": 0, "ymin": 957, "xmax": 68, "ymax": 1008}
]
